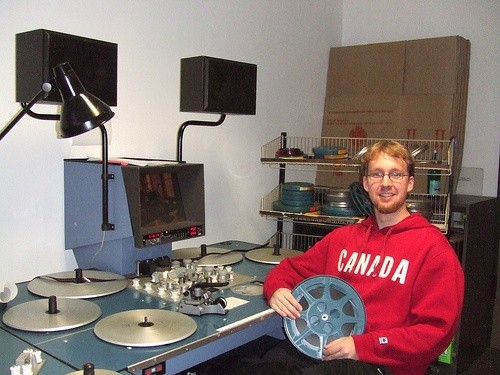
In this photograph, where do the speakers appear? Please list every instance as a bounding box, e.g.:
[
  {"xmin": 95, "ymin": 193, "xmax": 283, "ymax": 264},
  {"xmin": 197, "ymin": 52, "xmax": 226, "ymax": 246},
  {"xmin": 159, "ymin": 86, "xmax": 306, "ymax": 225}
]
[
  {"xmin": 181, "ymin": 57, "xmax": 257, "ymax": 116},
  {"xmin": 16, "ymin": 29, "xmax": 118, "ymax": 107}
]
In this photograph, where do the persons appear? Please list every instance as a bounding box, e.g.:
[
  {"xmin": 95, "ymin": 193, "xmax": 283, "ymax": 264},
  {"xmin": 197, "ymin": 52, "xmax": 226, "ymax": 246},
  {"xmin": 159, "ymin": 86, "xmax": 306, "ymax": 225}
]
[{"xmin": 264, "ymin": 141, "xmax": 464, "ymax": 375}]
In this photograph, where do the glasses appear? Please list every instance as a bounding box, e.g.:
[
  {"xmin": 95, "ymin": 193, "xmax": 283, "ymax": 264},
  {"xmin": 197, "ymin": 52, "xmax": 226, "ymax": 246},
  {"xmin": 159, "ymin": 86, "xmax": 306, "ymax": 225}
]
[{"xmin": 367, "ymin": 172, "xmax": 410, "ymax": 182}]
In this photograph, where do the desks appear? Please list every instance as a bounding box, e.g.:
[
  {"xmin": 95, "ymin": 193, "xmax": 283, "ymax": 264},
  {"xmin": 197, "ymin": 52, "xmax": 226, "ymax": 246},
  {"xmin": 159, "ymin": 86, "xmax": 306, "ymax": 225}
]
[{"xmin": 0, "ymin": 240, "xmax": 286, "ymax": 375}]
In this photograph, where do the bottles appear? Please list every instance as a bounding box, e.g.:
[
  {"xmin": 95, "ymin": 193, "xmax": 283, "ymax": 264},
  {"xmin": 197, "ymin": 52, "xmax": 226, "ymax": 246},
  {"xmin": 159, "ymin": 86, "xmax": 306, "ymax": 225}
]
[{"xmin": 428, "ymin": 153, "xmax": 440, "ymax": 199}]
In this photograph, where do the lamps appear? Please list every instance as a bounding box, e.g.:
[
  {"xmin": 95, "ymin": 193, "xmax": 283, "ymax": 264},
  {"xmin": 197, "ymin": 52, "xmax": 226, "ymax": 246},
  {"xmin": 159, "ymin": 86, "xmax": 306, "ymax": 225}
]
[{"xmin": 0, "ymin": 62, "xmax": 115, "ymax": 141}]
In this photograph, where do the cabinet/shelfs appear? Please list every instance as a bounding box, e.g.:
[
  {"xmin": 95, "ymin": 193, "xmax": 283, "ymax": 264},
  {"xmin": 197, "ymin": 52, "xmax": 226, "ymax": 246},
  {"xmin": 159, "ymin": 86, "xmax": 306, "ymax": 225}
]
[
  {"xmin": 294, "ymin": 194, "xmax": 499, "ymax": 374},
  {"xmin": 259, "ymin": 132, "xmax": 456, "ymax": 252}
]
